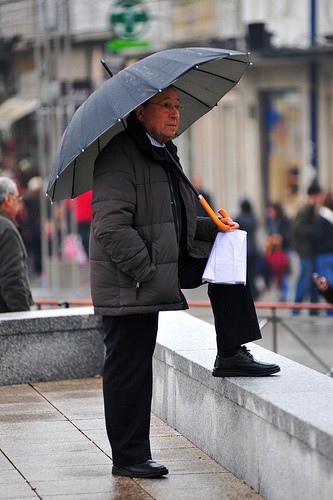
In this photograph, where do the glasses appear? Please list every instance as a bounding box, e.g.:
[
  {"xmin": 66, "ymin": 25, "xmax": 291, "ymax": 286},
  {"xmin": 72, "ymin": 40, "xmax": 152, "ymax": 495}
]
[
  {"xmin": 10, "ymin": 196, "xmax": 23, "ymax": 204},
  {"xmin": 150, "ymin": 101, "xmax": 184, "ymax": 113}
]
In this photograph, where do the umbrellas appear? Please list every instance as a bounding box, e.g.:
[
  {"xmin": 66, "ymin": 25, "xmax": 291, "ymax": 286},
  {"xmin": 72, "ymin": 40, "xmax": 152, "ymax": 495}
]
[{"xmin": 44, "ymin": 46, "xmax": 253, "ymax": 232}]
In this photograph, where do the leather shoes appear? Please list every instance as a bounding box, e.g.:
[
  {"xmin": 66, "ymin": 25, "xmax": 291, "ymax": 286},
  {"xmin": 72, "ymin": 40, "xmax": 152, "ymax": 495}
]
[
  {"xmin": 111, "ymin": 459, "xmax": 168, "ymax": 477},
  {"xmin": 212, "ymin": 345, "xmax": 280, "ymax": 377}
]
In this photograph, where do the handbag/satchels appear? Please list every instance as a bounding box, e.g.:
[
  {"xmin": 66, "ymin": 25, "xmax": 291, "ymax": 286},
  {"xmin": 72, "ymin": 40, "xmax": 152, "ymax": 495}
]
[{"xmin": 265, "ymin": 244, "xmax": 288, "ymax": 279}]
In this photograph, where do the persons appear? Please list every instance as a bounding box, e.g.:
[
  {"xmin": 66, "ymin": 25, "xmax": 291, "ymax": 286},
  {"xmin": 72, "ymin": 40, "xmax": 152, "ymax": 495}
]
[
  {"xmin": 89, "ymin": 84, "xmax": 281, "ymax": 478},
  {"xmin": 0, "ymin": 175, "xmax": 93, "ymax": 313},
  {"xmin": 183, "ymin": 166, "xmax": 333, "ymax": 317}
]
